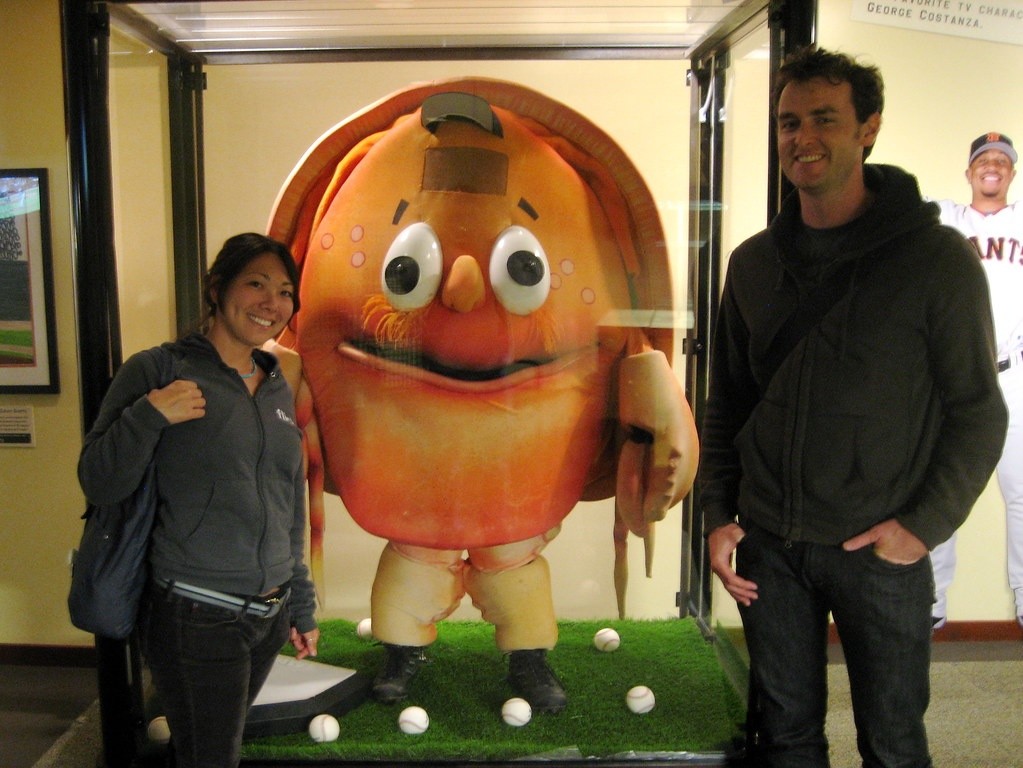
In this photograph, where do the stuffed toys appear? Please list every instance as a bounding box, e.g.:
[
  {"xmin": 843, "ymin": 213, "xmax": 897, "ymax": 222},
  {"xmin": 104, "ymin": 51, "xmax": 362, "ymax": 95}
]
[{"xmin": 260, "ymin": 76, "xmax": 701, "ymax": 711}]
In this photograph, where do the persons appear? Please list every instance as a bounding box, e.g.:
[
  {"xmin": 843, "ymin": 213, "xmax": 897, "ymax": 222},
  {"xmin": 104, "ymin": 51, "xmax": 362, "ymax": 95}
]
[
  {"xmin": 76, "ymin": 232, "xmax": 319, "ymax": 768},
  {"xmin": 920, "ymin": 131, "xmax": 1023, "ymax": 630},
  {"xmin": 703, "ymin": 44, "xmax": 1009, "ymax": 767}
]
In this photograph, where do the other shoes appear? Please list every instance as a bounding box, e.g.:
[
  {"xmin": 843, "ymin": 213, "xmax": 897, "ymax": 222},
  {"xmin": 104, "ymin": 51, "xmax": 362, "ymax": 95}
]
[
  {"xmin": 1015, "ymin": 615, "xmax": 1023, "ymax": 627},
  {"xmin": 932, "ymin": 616, "xmax": 947, "ymax": 627}
]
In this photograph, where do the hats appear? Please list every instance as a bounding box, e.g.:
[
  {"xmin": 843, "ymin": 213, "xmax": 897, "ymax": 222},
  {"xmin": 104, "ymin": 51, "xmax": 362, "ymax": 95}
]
[{"xmin": 969, "ymin": 132, "xmax": 1017, "ymax": 166}]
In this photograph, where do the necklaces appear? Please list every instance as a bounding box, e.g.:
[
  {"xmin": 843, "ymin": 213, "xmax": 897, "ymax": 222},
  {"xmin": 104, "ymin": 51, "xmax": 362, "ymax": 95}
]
[{"xmin": 240, "ymin": 358, "xmax": 258, "ymax": 378}]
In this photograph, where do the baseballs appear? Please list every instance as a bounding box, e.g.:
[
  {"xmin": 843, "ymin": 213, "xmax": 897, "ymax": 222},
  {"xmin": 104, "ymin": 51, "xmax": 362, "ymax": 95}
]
[
  {"xmin": 398, "ymin": 706, "xmax": 430, "ymax": 734},
  {"xmin": 594, "ymin": 628, "xmax": 621, "ymax": 652},
  {"xmin": 309, "ymin": 713, "xmax": 341, "ymax": 743},
  {"xmin": 356, "ymin": 618, "xmax": 373, "ymax": 639},
  {"xmin": 501, "ymin": 697, "xmax": 532, "ymax": 727},
  {"xmin": 626, "ymin": 685, "xmax": 656, "ymax": 715},
  {"xmin": 148, "ymin": 716, "xmax": 172, "ymax": 746}
]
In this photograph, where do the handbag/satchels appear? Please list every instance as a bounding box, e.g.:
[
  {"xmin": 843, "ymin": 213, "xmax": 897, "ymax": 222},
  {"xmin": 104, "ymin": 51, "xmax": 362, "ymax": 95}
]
[{"xmin": 69, "ymin": 346, "xmax": 174, "ymax": 637}]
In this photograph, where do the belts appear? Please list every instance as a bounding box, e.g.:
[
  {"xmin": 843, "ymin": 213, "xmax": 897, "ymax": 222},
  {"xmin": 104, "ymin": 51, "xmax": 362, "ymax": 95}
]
[
  {"xmin": 994, "ymin": 358, "xmax": 1011, "ymax": 374},
  {"xmin": 154, "ymin": 574, "xmax": 291, "ymax": 618}
]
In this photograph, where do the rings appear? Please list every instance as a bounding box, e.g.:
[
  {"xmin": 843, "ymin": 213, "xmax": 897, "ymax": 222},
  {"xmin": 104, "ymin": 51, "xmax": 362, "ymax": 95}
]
[{"xmin": 307, "ymin": 638, "xmax": 312, "ymax": 641}]
